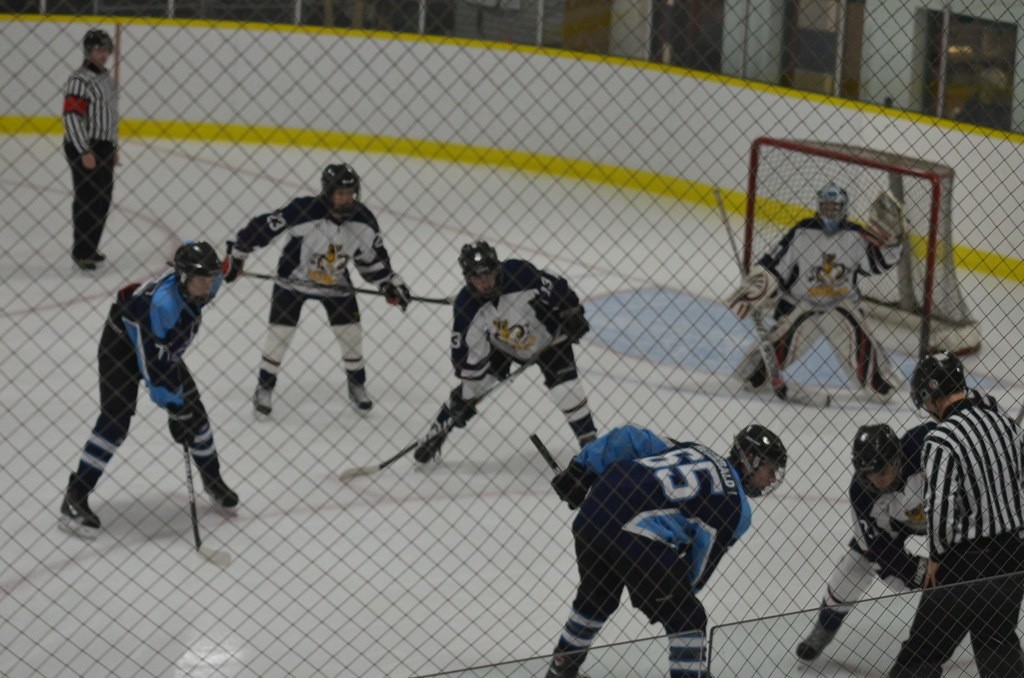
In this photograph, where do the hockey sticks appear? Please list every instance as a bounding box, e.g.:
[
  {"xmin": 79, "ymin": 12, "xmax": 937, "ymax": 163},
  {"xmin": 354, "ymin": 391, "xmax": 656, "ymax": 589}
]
[
  {"xmin": 1014, "ymin": 407, "xmax": 1024, "ymax": 425},
  {"xmin": 711, "ymin": 182, "xmax": 833, "ymax": 407},
  {"xmin": 179, "ymin": 440, "xmax": 234, "ymax": 566},
  {"xmin": 339, "ymin": 356, "xmax": 541, "ymax": 480},
  {"xmin": 237, "ymin": 269, "xmax": 465, "ymax": 304},
  {"xmin": 528, "ymin": 431, "xmax": 562, "ymax": 476}
]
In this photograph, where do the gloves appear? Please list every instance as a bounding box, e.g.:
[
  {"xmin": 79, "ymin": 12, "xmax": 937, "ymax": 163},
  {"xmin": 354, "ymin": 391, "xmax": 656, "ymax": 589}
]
[
  {"xmin": 550, "ymin": 461, "xmax": 593, "ymax": 511},
  {"xmin": 559, "ymin": 314, "xmax": 590, "ymax": 343},
  {"xmin": 218, "ymin": 242, "xmax": 252, "ymax": 284},
  {"xmin": 448, "ymin": 385, "xmax": 477, "ymax": 428},
  {"xmin": 167, "ymin": 409, "xmax": 195, "ymax": 448},
  {"xmin": 384, "ymin": 276, "xmax": 414, "ymax": 312}
]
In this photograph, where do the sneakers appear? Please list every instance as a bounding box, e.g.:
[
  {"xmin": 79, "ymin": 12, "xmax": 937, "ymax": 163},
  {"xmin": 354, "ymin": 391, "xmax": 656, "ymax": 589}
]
[
  {"xmin": 55, "ymin": 471, "xmax": 105, "ymax": 540},
  {"xmin": 347, "ymin": 369, "xmax": 376, "ymax": 418},
  {"xmin": 66, "ymin": 247, "xmax": 107, "ymax": 271},
  {"xmin": 795, "ymin": 606, "xmax": 848, "ymax": 671},
  {"xmin": 193, "ymin": 455, "xmax": 239, "ymax": 518},
  {"xmin": 411, "ymin": 410, "xmax": 455, "ymax": 473},
  {"xmin": 746, "ymin": 373, "xmax": 767, "ymax": 389},
  {"xmin": 864, "ymin": 385, "xmax": 904, "ymax": 405},
  {"xmin": 248, "ymin": 368, "xmax": 277, "ymax": 423}
]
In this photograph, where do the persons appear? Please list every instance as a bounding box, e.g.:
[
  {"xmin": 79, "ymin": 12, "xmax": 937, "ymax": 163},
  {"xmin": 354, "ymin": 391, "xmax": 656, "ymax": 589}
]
[
  {"xmin": 889, "ymin": 350, "xmax": 1024, "ymax": 678},
  {"xmin": 414, "ymin": 241, "xmax": 598, "ymax": 474},
  {"xmin": 726, "ymin": 183, "xmax": 906, "ymax": 406},
  {"xmin": 798, "ymin": 421, "xmax": 938, "ymax": 661},
  {"xmin": 955, "ymin": 69, "xmax": 1010, "ymax": 129},
  {"xmin": 546, "ymin": 423, "xmax": 786, "ymax": 678},
  {"xmin": 224, "ymin": 161, "xmax": 409, "ymax": 418},
  {"xmin": 63, "ymin": 29, "xmax": 119, "ymax": 271},
  {"xmin": 56, "ymin": 240, "xmax": 240, "ymax": 537}
]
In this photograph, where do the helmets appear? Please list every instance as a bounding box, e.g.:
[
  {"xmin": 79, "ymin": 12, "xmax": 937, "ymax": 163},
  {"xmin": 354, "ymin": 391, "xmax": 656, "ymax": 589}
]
[
  {"xmin": 83, "ymin": 29, "xmax": 113, "ymax": 54},
  {"xmin": 852, "ymin": 423, "xmax": 904, "ymax": 493},
  {"xmin": 174, "ymin": 241, "xmax": 224, "ymax": 306},
  {"xmin": 727, "ymin": 424, "xmax": 787, "ymax": 499},
  {"xmin": 909, "ymin": 349, "xmax": 966, "ymax": 408},
  {"xmin": 816, "ymin": 180, "xmax": 850, "ymax": 231},
  {"xmin": 321, "ymin": 164, "xmax": 361, "ymax": 217},
  {"xmin": 458, "ymin": 241, "xmax": 505, "ymax": 299}
]
